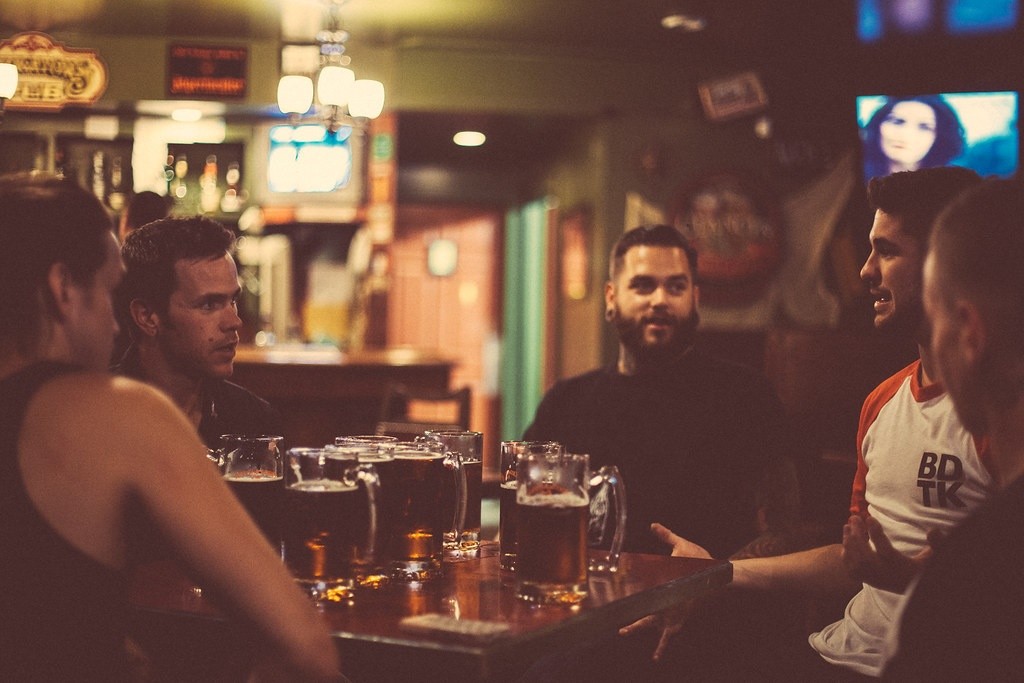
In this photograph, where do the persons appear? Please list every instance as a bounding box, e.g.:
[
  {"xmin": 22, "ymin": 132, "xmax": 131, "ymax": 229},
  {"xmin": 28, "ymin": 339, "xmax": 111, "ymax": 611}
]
[
  {"xmin": 861, "ymin": 95, "xmax": 980, "ymax": 178},
  {"xmin": 875, "ymin": 178, "xmax": 1023, "ymax": 683},
  {"xmin": 1, "ymin": 173, "xmax": 348, "ymax": 683},
  {"xmin": 109, "ymin": 189, "xmax": 288, "ymax": 461},
  {"xmin": 520, "ymin": 226, "xmax": 794, "ymax": 683},
  {"xmin": 619, "ymin": 166, "xmax": 993, "ymax": 683}
]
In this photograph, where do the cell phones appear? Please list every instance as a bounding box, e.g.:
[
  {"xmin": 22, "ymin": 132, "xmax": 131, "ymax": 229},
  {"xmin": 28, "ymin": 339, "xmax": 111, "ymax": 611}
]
[{"xmin": 399, "ymin": 613, "xmax": 510, "ymax": 647}]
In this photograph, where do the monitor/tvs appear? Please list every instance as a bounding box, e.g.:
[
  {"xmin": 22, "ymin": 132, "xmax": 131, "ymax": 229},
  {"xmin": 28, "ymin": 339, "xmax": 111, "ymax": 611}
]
[
  {"xmin": 246, "ymin": 121, "xmax": 365, "ymax": 210},
  {"xmin": 857, "ymin": 90, "xmax": 1019, "ymax": 181},
  {"xmin": 856, "ymin": 0, "xmax": 1018, "ymax": 40}
]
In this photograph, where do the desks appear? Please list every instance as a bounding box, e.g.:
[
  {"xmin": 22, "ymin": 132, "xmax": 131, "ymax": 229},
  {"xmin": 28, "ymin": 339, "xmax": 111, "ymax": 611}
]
[{"xmin": 128, "ymin": 550, "xmax": 733, "ymax": 683}]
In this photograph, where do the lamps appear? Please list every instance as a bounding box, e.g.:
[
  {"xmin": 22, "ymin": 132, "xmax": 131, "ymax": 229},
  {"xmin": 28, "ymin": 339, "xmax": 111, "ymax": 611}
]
[{"xmin": 278, "ymin": 0, "xmax": 384, "ymax": 135}]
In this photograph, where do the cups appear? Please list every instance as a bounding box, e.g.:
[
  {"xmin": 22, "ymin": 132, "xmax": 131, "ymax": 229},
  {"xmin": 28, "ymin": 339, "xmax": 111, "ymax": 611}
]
[
  {"xmin": 206, "ymin": 433, "xmax": 285, "ymax": 562},
  {"xmin": 287, "ymin": 427, "xmax": 485, "ymax": 592},
  {"xmin": 497, "ymin": 440, "xmax": 568, "ymax": 575},
  {"xmin": 515, "ymin": 455, "xmax": 627, "ymax": 603}
]
